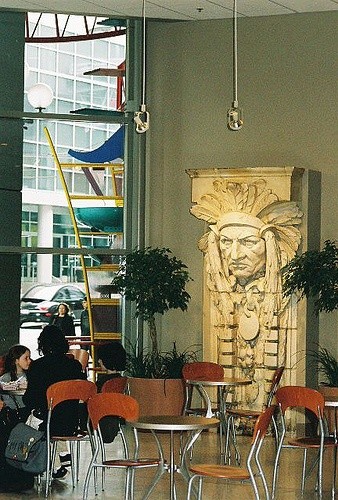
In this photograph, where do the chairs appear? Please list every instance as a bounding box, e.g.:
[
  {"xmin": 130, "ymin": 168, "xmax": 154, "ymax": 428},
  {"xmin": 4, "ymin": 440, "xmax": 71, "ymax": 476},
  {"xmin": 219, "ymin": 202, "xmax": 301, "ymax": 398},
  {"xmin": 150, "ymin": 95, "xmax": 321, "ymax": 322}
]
[{"xmin": 35, "ymin": 362, "xmax": 338, "ymax": 500}]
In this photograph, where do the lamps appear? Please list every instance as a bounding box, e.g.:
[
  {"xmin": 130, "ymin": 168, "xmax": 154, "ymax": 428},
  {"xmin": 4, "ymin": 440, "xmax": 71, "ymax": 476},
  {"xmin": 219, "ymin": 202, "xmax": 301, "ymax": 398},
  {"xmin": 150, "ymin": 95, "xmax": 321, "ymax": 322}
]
[
  {"xmin": 228, "ymin": 0, "xmax": 244, "ymax": 131},
  {"xmin": 134, "ymin": 0, "xmax": 150, "ymax": 134},
  {"xmin": 27, "ymin": 83, "xmax": 54, "ymax": 112}
]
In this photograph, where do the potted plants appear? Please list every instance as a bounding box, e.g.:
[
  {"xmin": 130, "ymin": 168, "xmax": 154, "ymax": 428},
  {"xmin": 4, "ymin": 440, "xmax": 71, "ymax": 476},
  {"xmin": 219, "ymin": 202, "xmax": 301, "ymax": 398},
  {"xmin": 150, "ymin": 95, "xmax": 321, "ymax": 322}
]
[
  {"xmin": 111, "ymin": 245, "xmax": 203, "ymax": 434},
  {"xmin": 280, "ymin": 238, "xmax": 338, "ymax": 436}
]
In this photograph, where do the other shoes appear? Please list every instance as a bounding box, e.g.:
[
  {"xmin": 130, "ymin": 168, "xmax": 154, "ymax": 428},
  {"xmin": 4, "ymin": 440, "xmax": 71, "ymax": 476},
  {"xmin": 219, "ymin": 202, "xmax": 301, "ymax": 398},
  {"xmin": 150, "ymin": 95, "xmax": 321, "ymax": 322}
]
[
  {"xmin": 52, "ymin": 465, "xmax": 69, "ymax": 480},
  {"xmin": 58, "ymin": 453, "xmax": 72, "ymax": 466}
]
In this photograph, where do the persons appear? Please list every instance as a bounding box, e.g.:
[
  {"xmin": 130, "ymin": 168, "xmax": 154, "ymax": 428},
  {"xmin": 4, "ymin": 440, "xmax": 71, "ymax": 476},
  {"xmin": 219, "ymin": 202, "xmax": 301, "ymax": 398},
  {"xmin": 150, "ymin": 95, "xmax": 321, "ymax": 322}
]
[
  {"xmin": 50, "ymin": 304, "xmax": 76, "ymax": 336},
  {"xmin": 0, "ymin": 325, "xmax": 82, "ymax": 480},
  {"xmin": 95, "ymin": 342, "xmax": 126, "ymax": 443},
  {"xmin": 189, "ymin": 180, "xmax": 303, "ymax": 369},
  {"xmin": 81, "ymin": 297, "xmax": 91, "ymax": 351}
]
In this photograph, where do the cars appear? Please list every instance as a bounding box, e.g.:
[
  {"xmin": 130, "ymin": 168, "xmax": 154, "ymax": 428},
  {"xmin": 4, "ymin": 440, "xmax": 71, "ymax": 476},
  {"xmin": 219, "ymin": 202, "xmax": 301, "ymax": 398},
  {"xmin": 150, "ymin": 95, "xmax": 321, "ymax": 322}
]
[{"xmin": 19, "ymin": 283, "xmax": 86, "ymax": 328}]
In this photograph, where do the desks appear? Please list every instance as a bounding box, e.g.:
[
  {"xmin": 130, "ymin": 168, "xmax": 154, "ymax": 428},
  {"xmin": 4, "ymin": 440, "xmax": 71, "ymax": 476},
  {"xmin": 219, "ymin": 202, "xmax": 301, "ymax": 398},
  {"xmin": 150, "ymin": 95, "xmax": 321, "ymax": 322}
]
[
  {"xmin": 186, "ymin": 377, "xmax": 252, "ymax": 454},
  {"xmin": 0, "ymin": 390, "xmax": 26, "ymax": 408},
  {"xmin": 125, "ymin": 416, "xmax": 220, "ymax": 500}
]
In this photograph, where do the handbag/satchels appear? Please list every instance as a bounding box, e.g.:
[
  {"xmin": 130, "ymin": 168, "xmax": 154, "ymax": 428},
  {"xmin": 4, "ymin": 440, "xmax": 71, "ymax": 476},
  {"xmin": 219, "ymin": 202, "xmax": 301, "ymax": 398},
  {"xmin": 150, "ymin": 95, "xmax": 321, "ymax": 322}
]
[{"xmin": 6, "ymin": 422, "xmax": 47, "ymax": 475}]
